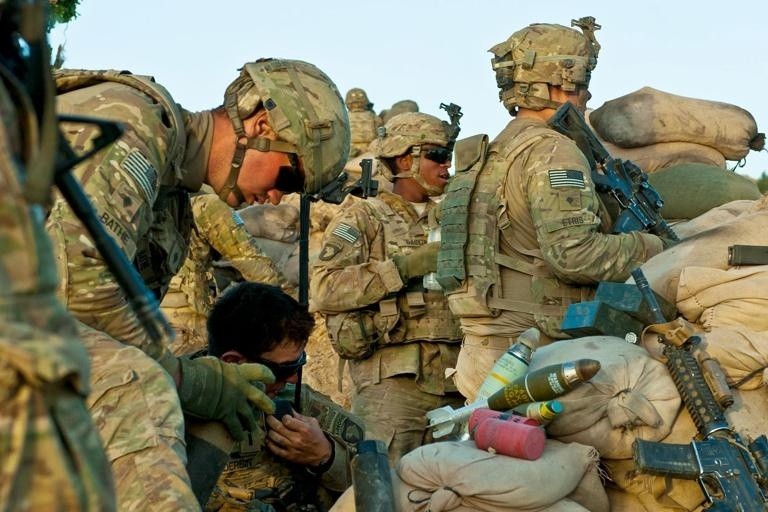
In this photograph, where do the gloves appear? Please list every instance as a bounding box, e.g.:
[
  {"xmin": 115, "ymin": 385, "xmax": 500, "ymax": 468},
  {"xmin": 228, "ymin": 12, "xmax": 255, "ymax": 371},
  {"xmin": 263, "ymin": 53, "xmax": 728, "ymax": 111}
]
[
  {"xmin": 176, "ymin": 356, "xmax": 277, "ymax": 442},
  {"xmin": 393, "ymin": 242, "xmax": 440, "ymax": 282}
]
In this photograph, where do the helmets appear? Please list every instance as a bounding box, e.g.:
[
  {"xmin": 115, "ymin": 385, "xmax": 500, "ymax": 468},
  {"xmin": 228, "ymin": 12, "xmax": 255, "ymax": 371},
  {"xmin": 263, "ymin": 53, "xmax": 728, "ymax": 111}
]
[
  {"xmin": 345, "ymin": 88, "xmax": 368, "ymax": 105},
  {"xmin": 375, "ymin": 112, "xmax": 454, "ymax": 181},
  {"xmin": 487, "ymin": 24, "xmax": 597, "ymax": 117},
  {"xmin": 237, "ymin": 60, "xmax": 350, "ymax": 193}
]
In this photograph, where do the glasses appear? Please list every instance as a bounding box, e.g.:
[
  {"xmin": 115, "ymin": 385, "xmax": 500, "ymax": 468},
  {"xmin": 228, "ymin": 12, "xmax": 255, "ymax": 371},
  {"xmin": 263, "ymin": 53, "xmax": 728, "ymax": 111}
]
[
  {"xmin": 421, "ymin": 147, "xmax": 452, "ymax": 164},
  {"xmin": 276, "ymin": 136, "xmax": 305, "ymax": 195},
  {"xmin": 241, "ymin": 350, "xmax": 305, "ymax": 382}
]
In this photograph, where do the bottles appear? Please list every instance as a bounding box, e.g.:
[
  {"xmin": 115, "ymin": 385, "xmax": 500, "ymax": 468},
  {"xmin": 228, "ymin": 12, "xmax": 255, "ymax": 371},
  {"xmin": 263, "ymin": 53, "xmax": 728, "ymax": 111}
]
[{"xmin": 421, "ymin": 227, "xmax": 445, "ymax": 290}]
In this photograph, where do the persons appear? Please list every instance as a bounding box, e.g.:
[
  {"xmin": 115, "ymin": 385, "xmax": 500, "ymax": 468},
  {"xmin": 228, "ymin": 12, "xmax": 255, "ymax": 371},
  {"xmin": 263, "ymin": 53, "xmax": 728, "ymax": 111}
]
[
  {"xmin": 0, "ymin": 1, "xmax": 117, "ymax": 512},
  {"xmin": 43, "ymin": 57, "xmax": 457, "ymax": 512},
  {"xmin": 436, "ymin": 21, "xmax": 674, "ymax": 402}
]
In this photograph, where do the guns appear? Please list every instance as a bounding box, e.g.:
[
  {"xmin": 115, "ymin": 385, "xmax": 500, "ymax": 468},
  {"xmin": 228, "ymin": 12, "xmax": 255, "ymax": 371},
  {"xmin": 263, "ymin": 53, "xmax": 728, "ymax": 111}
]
[
  {"xmin": 52, "ymin": 112, "xmax": 173, "ymax": 348},
  {"xmin": 629, "ymin": 266, "xmax": 768, "ymax": 510},
  {"xmin": 547, "ymin": 102, "xmax": 680, "ymax": 243}
]
